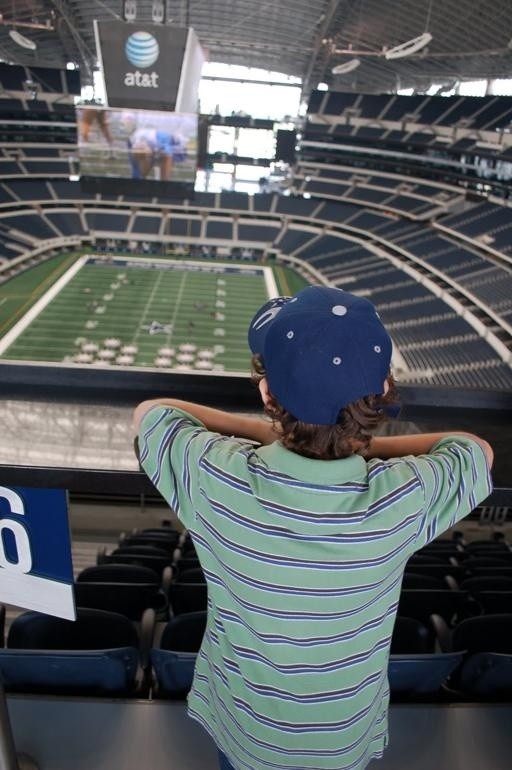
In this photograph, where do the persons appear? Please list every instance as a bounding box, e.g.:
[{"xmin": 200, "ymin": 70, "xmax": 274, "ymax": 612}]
[
  {"xmin": 128, "ymin": 129, "xmax": 188, "ymax": 180},
  {"xmin": 131, "ymin": 288, "xmax": 496, "ymax": 770},
  {"xmin": 77, "ymin": 107, "xmax": 116, "ymax": 161}
]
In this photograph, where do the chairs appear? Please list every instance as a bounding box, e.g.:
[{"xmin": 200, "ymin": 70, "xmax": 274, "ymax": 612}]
[
  {"xmin": 1, "ymin": 61, "xmax": 511, "ymax": 388},
  {"xmin": 1, "ymin": 515, "xmax": 512, "ymax": 769}
]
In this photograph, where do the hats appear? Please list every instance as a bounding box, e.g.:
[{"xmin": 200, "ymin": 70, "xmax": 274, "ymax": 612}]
[{"xmin": 248, "ymin": 286, "xmax": 392, "ymax": 424}]
